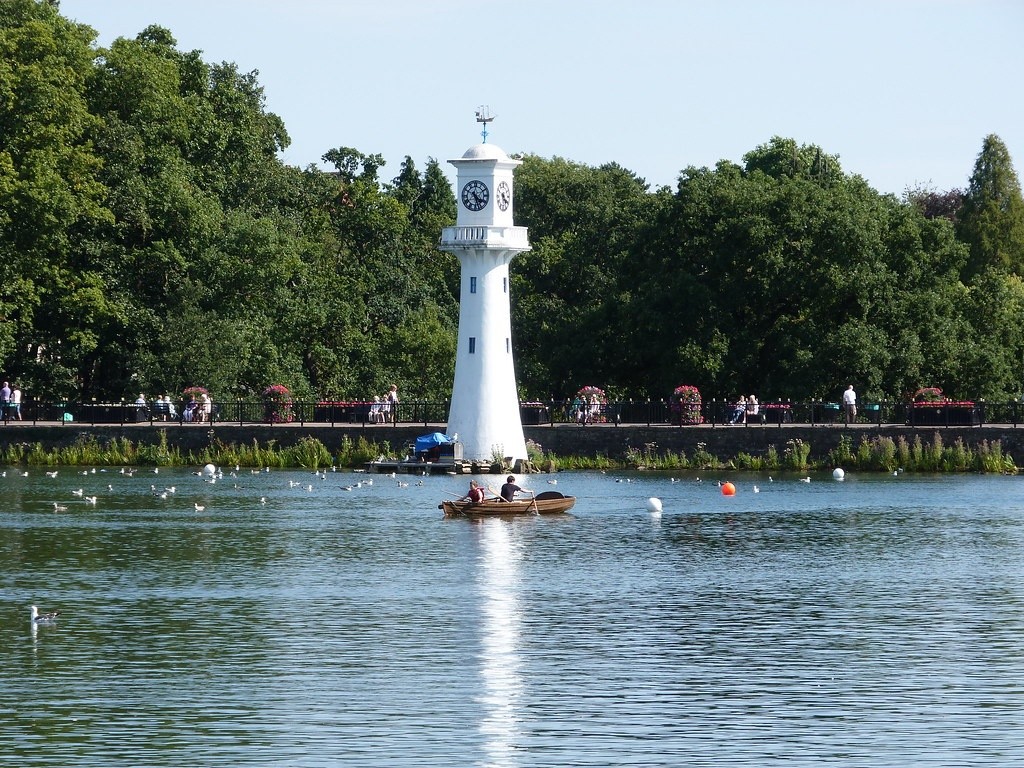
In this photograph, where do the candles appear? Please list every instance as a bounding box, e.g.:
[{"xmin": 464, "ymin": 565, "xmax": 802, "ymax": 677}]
[{"xmin": 355, "ymin": 398, "xmax": 358, "ymax": 401}]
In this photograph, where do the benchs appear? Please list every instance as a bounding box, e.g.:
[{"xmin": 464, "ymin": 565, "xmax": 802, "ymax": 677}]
[
  {"xmin": 721, "ymin": 403, "xmax": 769, "ymax": 425},
  {"xmin": 147, "ymin": 403, "xmax": 170, "ymax": 422},
  {"xmin": 349, "ymin": 408, "xmax": 388, "ymax": 424}
]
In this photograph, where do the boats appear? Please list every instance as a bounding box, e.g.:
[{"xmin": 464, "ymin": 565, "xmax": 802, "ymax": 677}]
[{"xmin": 438, "ymin": 489, "xmax": 577, "ymax": 518}]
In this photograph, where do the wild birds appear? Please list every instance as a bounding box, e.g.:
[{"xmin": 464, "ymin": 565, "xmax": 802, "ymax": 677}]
[
  {"xmin": 799, "ymin": 477, "xmax": 812, "ymax": 483},
  {"xmin": 615, "ymin": 478, "xmax": 623, "ymax": 483},
  {"xmin": 768, "ymin": 475, "xmax": 774, "ymax": 481},
  {"xmin": 547, "ymin": 479, "xmax": 557, "ymax": 485},
  {"xmin": 670, "ymin": 478, "xmax": 681, "ymax": 484},
  {"xmin": 752, "ymin": 486, "xmax": 760, "ymax": 493},
  {"xmin": 696, "ymin": 476, "xmax": 703, "ymax": 483},
  {"xmin": 1, "ymin": 465, "xmax": 424, "ymax": 513},
  {"xmin": 712, "ymin": 480, "xmax": 729, "ymax": 487}
]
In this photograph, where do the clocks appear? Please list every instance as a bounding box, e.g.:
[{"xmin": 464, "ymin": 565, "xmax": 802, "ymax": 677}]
[
  {"xmin": 496, "ymin": 181, "xmax": 511, "ymax": 212},
  {"xmin": 462, "ymin": 180, "xmax": 489, "ymax": 211}
]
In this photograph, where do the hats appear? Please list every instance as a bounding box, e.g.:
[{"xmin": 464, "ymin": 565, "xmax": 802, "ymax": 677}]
[
  {"xmin": 580, "ymin": 395, "xmax": 586, "ymax": 400},
  {"xmin": 164, "ymin": 396, "xmax": 170, "ymax": 400},
  {"xmin": 382, "ymin": 394, "xmax": 388, "ymax": 399},
  {"xmin": 592, "ymin": 394, "xmax": 596, "ymax": 398},
  {"xmin": 373, "ymin": 396, "xmax": 379, "ymax": 401}
]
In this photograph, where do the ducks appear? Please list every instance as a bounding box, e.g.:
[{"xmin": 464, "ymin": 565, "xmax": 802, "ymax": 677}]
[{"xmin": 26, "ymin": 605, "xmax": 61, "ymax": 621}]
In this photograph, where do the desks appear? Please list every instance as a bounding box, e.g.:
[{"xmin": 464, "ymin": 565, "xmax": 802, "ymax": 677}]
[
  {"xmin": 313, "ymin": 405, "xmax": 373, "ymax": 423},
  {"xmin": 906, "ymin": 405, "xmax": 972, "ymax": 426},
  {"xmin": 82, "ymin": 405, "xmax": 147, "ymax": 422},
  {"xmin": 521, "ymin": 405, "xmax": 549, "ymax": 425}
]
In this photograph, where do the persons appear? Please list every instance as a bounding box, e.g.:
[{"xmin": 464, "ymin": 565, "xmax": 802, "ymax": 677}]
[
  {"xmin": 155, "ymin": 395, "xmax": 164, "ymax": 411},
  {"xmin": 135, "ymin": 394, "xmax": 145, "ymax": 403},
  {"xmin": 843, "ymin": 385, "xmax": 857, "ymax": 422},
  {"xmin": 742, "ymin": 394, "xmax": 758, "ymax": 423},
  {"xmin": 499, "ymin": 476, "xmax": 534, "ymax": 502},
  {"xmin": 576, "ymin": 394, "xmax": 599, "ymax": 425},
  {"xmin": 0, "ymin": 382, "xmax": 11, "ymax": 419},
  {"xmin": 386, "ymin": 384, "xmax": 399, "ymax": 421},
  {"xmin": 369, "ymin": 395, "xmax": 381, "ymax": 424},
  {"xmin": 376, "ymin": 394, "xmax": 390, "ymax": 424},
  {"xmin": 164, "ymin": 396, "xmax": 180, "ymax": 420},
  {"xmin": 729, "ymin": 395, "xmax": 746, "ymax": 423},
  {"xmin": 183, "ymin": 394, "xmax": 198, "ymax": 422},
  {"xmin": 197, "ymin": 394, "xmax": 211, "ymax": 422},
  {"xmin": 462, "ymin": 480, "xmax": 483, "ymax": 502},
  {"xmin": 10, "ymin": 384, "xmax": 22, "ymax": 420}
]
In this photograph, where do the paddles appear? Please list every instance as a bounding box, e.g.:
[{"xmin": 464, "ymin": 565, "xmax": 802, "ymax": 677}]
[
  {"xmin": 487, "ymin": 485, "xmax": 510, "ymax": 503},
  {"xmin": 531, "ymin": 490, "xmax": 539, "ymax": 515}
]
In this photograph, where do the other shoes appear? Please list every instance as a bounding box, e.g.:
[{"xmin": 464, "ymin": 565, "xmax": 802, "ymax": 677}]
[
  {"xmin": 152, "ymin": 417, "xmax": 157, "ymax": 420},
  {"xmin": 742, "ymin": 421, "xmax": 746, "ymax": 423},
  {"xmin": 730, "ymin": 420, "xmax": 735, "ymax": 424},
  {"xmin": 145, "ymin": 418, "xmax": 148, "ymax": 421},
  {"xmin": 370, "ymin": 421, "xmax": 372, "ymax": 424},
  {"xmin": 373, "ymin": 421, "xmax": 376, "ymax": 424},
  {"xmin": 576, "ymin": 422, "xmax": 579, "ymax": 425},
  {"xmin": 590, "ymin": 421, "xmax": 593, "ymax": 425},
  {"xmin": 584, "ymin": 422, "xmax": 587, "ymax": 425}
]
